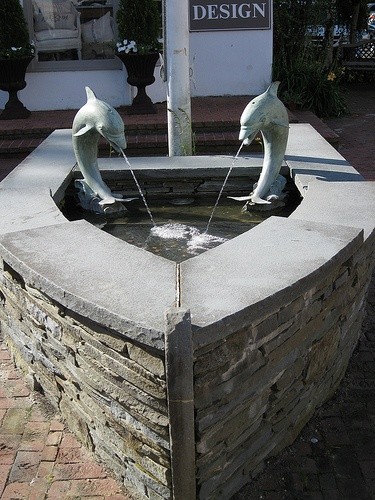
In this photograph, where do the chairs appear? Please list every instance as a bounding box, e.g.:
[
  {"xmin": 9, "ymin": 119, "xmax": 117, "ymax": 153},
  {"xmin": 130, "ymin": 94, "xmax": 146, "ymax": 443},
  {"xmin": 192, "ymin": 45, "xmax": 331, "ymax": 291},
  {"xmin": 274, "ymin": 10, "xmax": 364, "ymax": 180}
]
[{"xmin": 32, "ymin": 0, "xmax": 82, "ymax": 61}]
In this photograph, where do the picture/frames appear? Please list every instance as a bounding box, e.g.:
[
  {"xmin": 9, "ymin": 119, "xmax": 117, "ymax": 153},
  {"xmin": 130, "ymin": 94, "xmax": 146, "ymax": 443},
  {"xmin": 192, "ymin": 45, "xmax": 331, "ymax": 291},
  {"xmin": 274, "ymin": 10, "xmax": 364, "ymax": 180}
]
[{"xmin": 23, "ymin": 0, "xmax": 123, "ymax": 73}]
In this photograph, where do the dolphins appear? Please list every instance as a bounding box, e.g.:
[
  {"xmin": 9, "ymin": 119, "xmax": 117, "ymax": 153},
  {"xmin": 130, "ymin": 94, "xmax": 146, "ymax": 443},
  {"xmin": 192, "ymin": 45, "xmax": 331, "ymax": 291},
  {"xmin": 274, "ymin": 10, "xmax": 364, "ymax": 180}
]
[
  {"xmin": 225, "ymin": 80, "xmax": 290, "ymax": 207},
  {"xmin": 70, "ymin": 86, "xmax": 127, "ymax": 204}
]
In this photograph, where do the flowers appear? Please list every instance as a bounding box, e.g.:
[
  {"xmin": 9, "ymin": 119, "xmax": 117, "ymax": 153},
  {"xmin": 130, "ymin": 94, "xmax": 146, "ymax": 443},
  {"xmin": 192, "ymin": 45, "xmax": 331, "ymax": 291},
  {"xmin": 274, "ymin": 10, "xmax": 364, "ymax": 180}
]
[
  {"xmin": 116, "ymin": 35, "xmax": 156, "ymax": 54},
  {"xmin": 0, "ymin": 40, "xmax": 36, "ymax": 58}
]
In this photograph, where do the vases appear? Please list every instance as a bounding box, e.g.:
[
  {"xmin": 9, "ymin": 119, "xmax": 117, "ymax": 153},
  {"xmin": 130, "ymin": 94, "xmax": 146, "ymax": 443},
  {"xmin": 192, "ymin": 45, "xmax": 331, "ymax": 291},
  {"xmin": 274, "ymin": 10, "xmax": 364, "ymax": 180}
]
[
  {"xmin": 117, "ymin": 53, "xmax": 160, "ymax": 113},
  {"xmin": 0, "ymin": 57, "xmax": 34, "ymax": 120}
]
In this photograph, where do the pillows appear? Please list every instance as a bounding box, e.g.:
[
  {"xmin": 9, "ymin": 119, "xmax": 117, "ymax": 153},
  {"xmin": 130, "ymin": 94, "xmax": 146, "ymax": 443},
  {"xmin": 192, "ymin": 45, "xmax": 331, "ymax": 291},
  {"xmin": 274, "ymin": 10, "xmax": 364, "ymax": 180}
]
[{"xmin": 81, "ymin": 12, "xmax": 114, "ymax": 42}]
